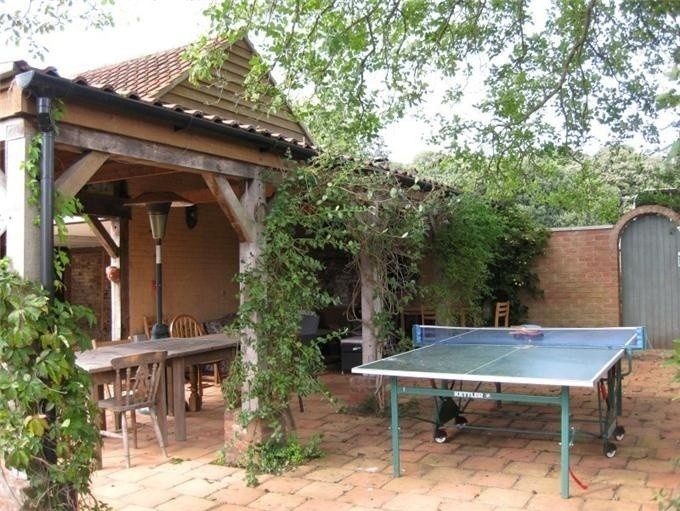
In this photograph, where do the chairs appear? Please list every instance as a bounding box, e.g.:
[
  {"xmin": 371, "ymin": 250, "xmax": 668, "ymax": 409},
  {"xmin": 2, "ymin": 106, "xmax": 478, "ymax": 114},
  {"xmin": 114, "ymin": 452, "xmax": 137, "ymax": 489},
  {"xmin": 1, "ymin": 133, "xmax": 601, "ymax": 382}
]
[
  {"xmin": 495, "ymin": 301, "xmax": 510, "ymax": 327},
  {"xmin": 420, "ymin": 303, "xmax": 438, "ymax": 325},
  {"xmin": 144, "ymin": 316, "xmax": 154, "ymax": 340},
  {"xmin": 169, "ymin": 314, "xmax": 223, "ymax": 397},
  {"xmin": 91, "ymin": 335, "xmax": 134, "ymax": 429},
  {"xmin": 92, "ymin": 350, "xmax": 168, "ymax": 469}
]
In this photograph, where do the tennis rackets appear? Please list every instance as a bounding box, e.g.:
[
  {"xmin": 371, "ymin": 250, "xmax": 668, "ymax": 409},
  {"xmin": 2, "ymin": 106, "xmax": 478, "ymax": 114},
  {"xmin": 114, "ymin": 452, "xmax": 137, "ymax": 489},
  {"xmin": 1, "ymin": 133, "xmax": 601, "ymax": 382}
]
[{"xmin": 509, "ymin": 324, "xmax": 542, "ymax": 337}]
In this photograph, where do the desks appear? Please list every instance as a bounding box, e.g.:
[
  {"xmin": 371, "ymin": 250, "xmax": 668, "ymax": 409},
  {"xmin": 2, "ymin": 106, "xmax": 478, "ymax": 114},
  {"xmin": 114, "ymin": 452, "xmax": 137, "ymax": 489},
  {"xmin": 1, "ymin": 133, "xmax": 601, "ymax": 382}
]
[
  {"xmin": 351, "ymin": 324, "xmax": 645, "ymax": 499},
  {"xmin": 75, "ymin": 332, "xmax": 240, "ymax": 472},
  {"xmin": 399, "ymin": 305, "xmax": 466, "ymax": 340}
]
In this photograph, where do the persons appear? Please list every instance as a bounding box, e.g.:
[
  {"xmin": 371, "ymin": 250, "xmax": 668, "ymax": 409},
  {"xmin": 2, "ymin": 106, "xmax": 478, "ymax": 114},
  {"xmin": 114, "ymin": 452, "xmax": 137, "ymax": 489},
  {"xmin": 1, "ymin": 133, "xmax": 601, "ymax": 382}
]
[{"xmin": 105, "ymin": 265, "xmax": 122, "ymax": 283}]
